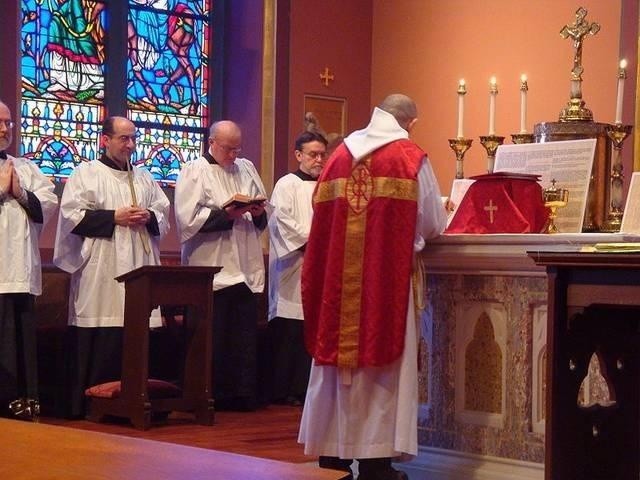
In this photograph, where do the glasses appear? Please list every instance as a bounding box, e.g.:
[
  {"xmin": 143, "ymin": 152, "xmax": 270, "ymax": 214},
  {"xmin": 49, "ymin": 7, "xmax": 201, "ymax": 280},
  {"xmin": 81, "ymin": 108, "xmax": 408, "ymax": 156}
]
[
  {"xmin": 104, "ymin": 132, "xmax": 138, "ymax": 144},
  {"xmin": 212, "ymin": 138, "xmax": 242, "ymax": 154},
  {"xmin": 0, "ymin": 119, "xmax": 17, "ymax": 129},
  {"xmin": 300, "ymin": 149, "xmax": 330, "ymax": 160}
]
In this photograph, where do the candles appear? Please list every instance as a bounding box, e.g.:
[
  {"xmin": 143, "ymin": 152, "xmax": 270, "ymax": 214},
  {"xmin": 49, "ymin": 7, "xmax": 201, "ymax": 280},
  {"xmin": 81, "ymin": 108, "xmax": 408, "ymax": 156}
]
[
  {"xmin": 457, "ymin": 79, "xmax": 467, "ymax": 139},
  {"xmin": 615, "ymin": 59, "xmax": 628, "ymax": 124},
  {"xmin": 519, "ymin": 74, "xmax": 529, "ymax": 134},
  {"xmin": 488, "ymin": 76, "xmax": 498, "ymax": 136}
]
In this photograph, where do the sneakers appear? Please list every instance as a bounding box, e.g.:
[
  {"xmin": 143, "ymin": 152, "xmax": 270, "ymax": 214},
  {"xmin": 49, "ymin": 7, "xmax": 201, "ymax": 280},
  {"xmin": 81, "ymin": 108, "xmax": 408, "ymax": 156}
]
[
  {"xmin": 208, "ymin": 389, "xmax": 313, "ymax": 413},
  {"xmin": 358, "ymin": 465, "xmax": 409, "ymax": 480},
  {"xmin": 317, "ymin": 454, "xmax": 355, "ymax": 469}
]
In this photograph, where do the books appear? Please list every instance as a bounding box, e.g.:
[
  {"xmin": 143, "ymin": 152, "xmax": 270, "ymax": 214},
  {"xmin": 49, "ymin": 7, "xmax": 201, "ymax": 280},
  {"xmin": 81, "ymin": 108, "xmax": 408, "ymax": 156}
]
[
  {"xmin": 222, "ymin": 193, "xmax": 266, "ymax": 211},
  {"xmin": 466, "ymin": 171, "xmax": 544, "ymax": 184}
]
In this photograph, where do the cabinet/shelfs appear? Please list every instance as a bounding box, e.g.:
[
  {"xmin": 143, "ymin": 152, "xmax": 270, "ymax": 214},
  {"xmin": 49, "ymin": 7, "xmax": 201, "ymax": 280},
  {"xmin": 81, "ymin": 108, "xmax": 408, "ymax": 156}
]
[{"xmin": 526, "ymin": 249, "xmax": 640, "ymax": 480}]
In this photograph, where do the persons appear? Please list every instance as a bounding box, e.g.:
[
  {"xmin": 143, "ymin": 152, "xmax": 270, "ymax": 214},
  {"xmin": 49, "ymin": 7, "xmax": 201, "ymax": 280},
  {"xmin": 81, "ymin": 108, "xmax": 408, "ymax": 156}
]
[
  {"xmin": 52, "ymin": 113, "xmax": 172, "ymax": 422},
  {"xmin": 296, "ymin": 91, "xmax": 450, "ymax": 480},
  {"xmin": 266, "ymin": 129, "xmax": 331, "ymax": 408},
  {"xmin": 0, "ymin": 102, "xmax": 60, "ymax": 424},
  {"xmin": 172, "ymin": 117, "xmax": 274, "ymax": 413}
]
